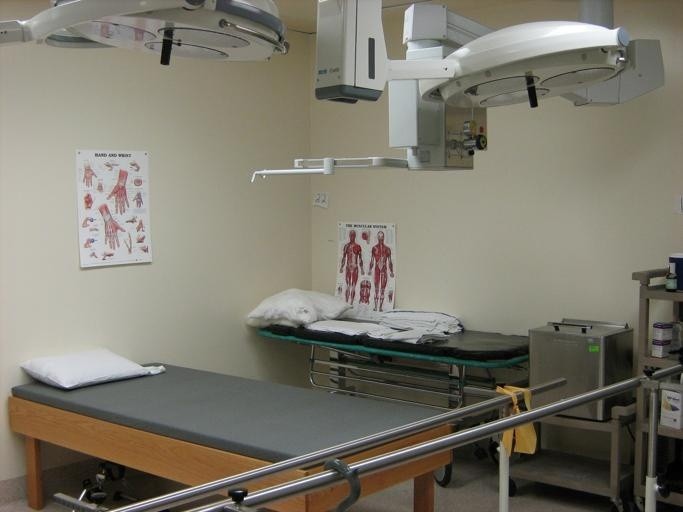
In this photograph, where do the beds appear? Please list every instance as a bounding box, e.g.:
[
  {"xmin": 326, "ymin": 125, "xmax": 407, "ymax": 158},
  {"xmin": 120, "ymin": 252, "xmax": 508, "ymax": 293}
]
[
  {"xmin": 247, "ymin": 303, "xmax": 532, "ymax": 489},
  {"xmin": 7, "ymin": 360, "xmax": 454, "ymax": 512}
]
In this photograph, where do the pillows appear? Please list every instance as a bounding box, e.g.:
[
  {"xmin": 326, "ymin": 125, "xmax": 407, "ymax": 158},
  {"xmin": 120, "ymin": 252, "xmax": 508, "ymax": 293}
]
[
  {"xmin": 22, "ymin": 347, "xmax": 169, "ymax": 393},
  {"xmin": 248, "ymin": 285, "xmax": 355, "ymax": 329}
]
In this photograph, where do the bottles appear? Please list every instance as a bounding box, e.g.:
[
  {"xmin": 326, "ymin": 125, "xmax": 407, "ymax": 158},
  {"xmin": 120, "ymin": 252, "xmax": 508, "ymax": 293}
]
[{"xmin": 666, "ymin": 262, "xmax": 677, "ymax": 293}]
